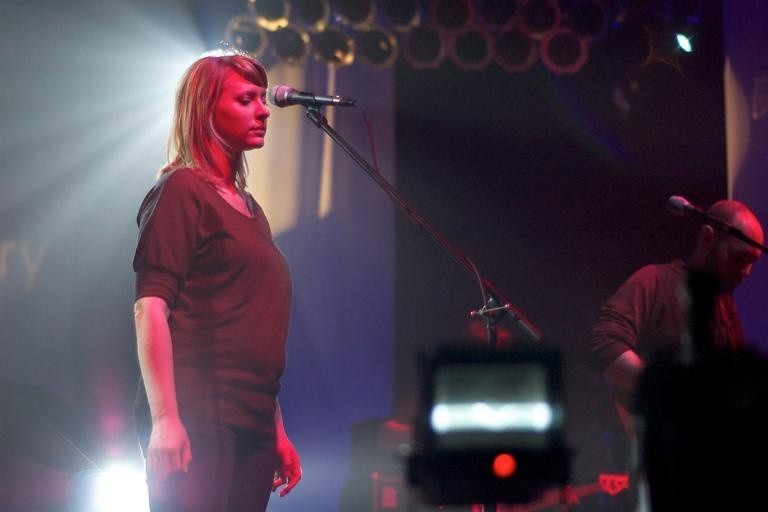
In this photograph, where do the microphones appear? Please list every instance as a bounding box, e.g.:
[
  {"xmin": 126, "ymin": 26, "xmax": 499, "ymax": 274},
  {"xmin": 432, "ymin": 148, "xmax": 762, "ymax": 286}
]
[
  {"xmin": 667, "ymin": 194, "xmax": 732, "ymax": 232},
  {"xmin": 268, "ymin": 85, "xmax": 352, "ymax": 107}
]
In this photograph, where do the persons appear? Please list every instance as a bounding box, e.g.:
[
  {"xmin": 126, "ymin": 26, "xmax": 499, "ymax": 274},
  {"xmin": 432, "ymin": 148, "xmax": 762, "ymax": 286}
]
[
  {"xmin": 129, "ymin": 49, "xmax": 304, "ymax": 511},
  {"xmin": 583, "ymin": 197, "xmax": 766, "ymax": 511}
]
